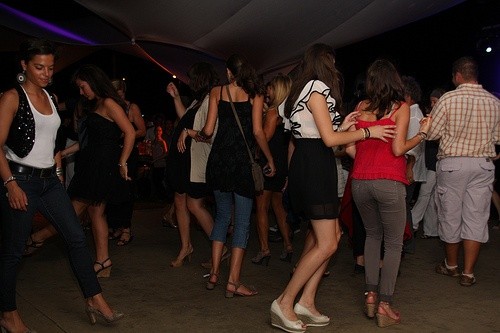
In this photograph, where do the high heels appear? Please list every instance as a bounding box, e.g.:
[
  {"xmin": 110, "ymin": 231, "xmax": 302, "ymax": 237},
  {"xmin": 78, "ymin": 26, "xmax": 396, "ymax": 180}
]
[
  {"xmin": 271, "ymin": 300, "xmax": 306, "ymax": 333},
  {"xmin": 94, "ymin": 256, "xmax": 113, "ymax": 279},
  {"xmin": 171, "ymin": 244, "xmax": 194, "ymax": 267},
  {"xmin": 278, "ymin": 244, "xmax": 295, "ymax": 264},
  {"xmin": 251, "ymin": 249, "xmax": 271, "ymax": 265},
  {"xmin": 86, "ymin": 305, "xmax": 126, "ymax": 325},
  {"xmin": 375, "ymin": 302, "xmax": 401, "ymax": 328},
  {"xmin": 364, "ymin": 291, "xmax": 378, "ymax": 319},
  {"xmin": 161, "ymin": 214, "xmax": 179, "ymax": 229},
  {"xmin": 202, "ymin": 252, "xmax": 231, "ymax": 269},
  {"xmin": 294, "ymin": 302, "xmax": 331, "ymax": 328},
  {"xmin": 24, "ymin": 231, "xmax": 44, "ymax": 255},
  {"xmin": 1, "ymin": 320, "xmax": 38, "ymax": 333}
]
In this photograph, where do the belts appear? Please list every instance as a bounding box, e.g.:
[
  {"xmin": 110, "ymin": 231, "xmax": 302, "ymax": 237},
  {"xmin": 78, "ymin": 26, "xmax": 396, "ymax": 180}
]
[{"xmin": 8, "ymin": 159, "xmax": 58, "ymax": 180}]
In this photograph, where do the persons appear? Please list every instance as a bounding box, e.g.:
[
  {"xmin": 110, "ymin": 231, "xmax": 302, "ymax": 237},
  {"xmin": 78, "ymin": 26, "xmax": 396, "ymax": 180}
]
[
  {"xmin": 0, "ymin": 37, "xmax": 172, "ymax": 332},
  {"xmin": 167, "ymin": 43, "xmax": 499, "ymax": 333}
]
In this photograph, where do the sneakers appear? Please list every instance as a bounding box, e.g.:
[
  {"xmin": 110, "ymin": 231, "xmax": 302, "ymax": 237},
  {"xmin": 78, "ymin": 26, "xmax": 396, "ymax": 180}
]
[
  {"xmin": 460, "ymin": 272, "xmax": 478, "ymax": 286},
  {"xmin": 379, "ymin": 266, "xmax": 401, "ymax": 278},
  {"xmin": 434, "ymin": 260, "xmax": 461, "ymax": 279},
  {"xmin": 353, "ymin": 262, "xmax": 364, "ymax": 274}
]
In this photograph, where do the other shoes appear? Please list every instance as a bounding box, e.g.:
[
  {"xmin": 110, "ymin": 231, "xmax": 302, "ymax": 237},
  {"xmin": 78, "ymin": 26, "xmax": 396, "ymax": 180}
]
[
  {"xmin": 402, "ymin": 231, "xmax": 415, "ymax": 254},
  {"xmin": 266, "ymin": 228, "xmax": 294, "ymax": 244},
  {"xmin": 419, "ymin": 233, "xmax": 439, "ymax": 239},
  {"xmin": 291, "ymin": 226, "xmax": 301, "ymax": 235},
  {"xmin": 268, "ymin": 221, "xmax": 281, "ymax": 234}
]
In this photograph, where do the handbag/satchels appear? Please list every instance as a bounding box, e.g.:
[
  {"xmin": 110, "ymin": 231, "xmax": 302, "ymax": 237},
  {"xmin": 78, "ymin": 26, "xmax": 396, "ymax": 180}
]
[{"xmin": 250, "ymin": 158, "xmax": 265, "ymax": 191}]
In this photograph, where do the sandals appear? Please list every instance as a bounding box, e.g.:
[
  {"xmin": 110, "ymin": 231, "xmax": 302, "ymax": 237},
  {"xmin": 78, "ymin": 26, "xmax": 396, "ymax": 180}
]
[
  {"xmin": 207, "ymin": 272, "xmax": 217, "ymax": 291},
  {"xmin": 108, "ymin": 230, "xmax": 122, "ymax": 241},
  {"xmin": 116, "ymin": 232, "xmax": 134, "ymax": 246},
  {"xmin": 224, "ymin": 280, "xmax": 258, "ymax": 299}
]
